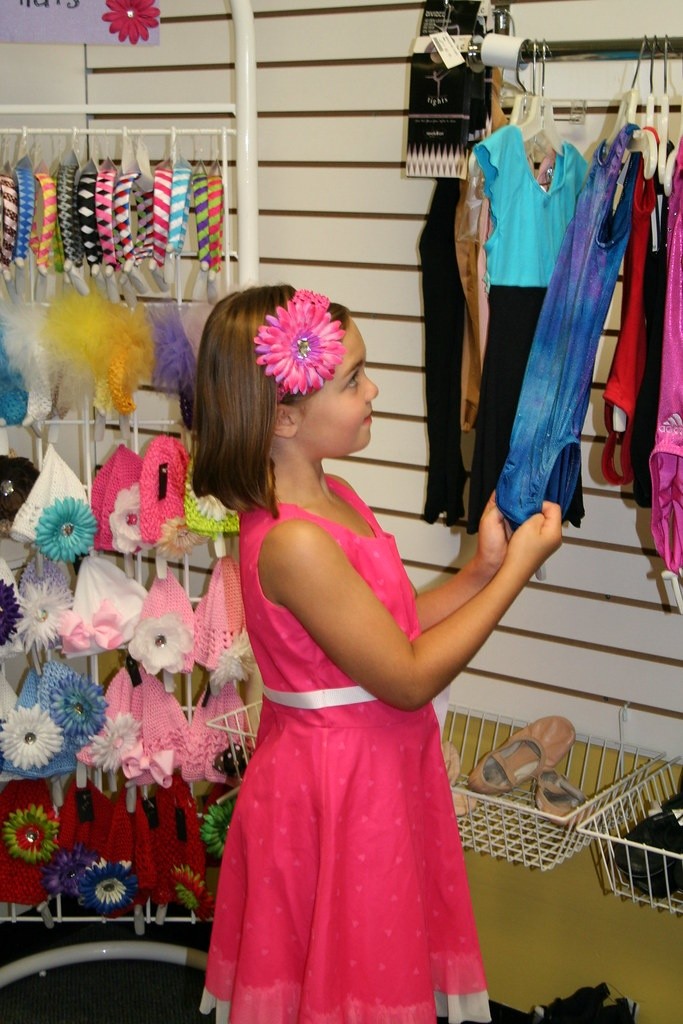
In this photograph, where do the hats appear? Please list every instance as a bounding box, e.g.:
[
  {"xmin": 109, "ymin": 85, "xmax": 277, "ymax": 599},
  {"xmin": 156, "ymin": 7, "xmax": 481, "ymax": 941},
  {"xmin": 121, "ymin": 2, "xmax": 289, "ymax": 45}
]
[{"xmin": 0, "ymin": 433, "xmax": 258, "ymax": 923}]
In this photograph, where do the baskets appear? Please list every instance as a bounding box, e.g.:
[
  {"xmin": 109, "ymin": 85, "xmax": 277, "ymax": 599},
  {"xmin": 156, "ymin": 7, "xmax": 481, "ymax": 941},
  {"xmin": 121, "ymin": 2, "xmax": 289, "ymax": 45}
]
[
  {"xmin": 206, "ymin": 688, "xmax": 667, "ymax": 871},
  {"xmin": 577, "ymin": 755, "xmax": 683, "ymax": 916}
]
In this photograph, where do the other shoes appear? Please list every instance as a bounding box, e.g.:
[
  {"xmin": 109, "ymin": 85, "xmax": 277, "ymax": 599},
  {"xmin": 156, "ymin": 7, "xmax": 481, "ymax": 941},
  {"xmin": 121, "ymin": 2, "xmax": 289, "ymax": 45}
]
[
  {"xmin": 466, "ymin": 716, "xmax": 575, "ymax": 796},
  {"xmin": 536, "ymin": 768, "xmax": 588, "ymax": 825}
]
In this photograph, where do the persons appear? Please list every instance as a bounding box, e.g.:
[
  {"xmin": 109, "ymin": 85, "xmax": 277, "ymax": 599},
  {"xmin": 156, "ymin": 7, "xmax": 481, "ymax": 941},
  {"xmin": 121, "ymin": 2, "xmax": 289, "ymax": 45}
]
[{"xmin": 192, "ymin": 287, "xmax": 565, "ymax": 1024}]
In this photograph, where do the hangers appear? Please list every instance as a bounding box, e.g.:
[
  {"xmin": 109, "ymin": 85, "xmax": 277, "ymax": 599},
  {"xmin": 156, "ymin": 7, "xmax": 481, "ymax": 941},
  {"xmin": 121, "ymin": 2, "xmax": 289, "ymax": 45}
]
[
  {"xmin": 599, "ymin": 34, "xmax": 683, "ymax": 196},
  {"xmin": 468, "ymin": 38, "xmax": 565, "ymax": 175}
]
